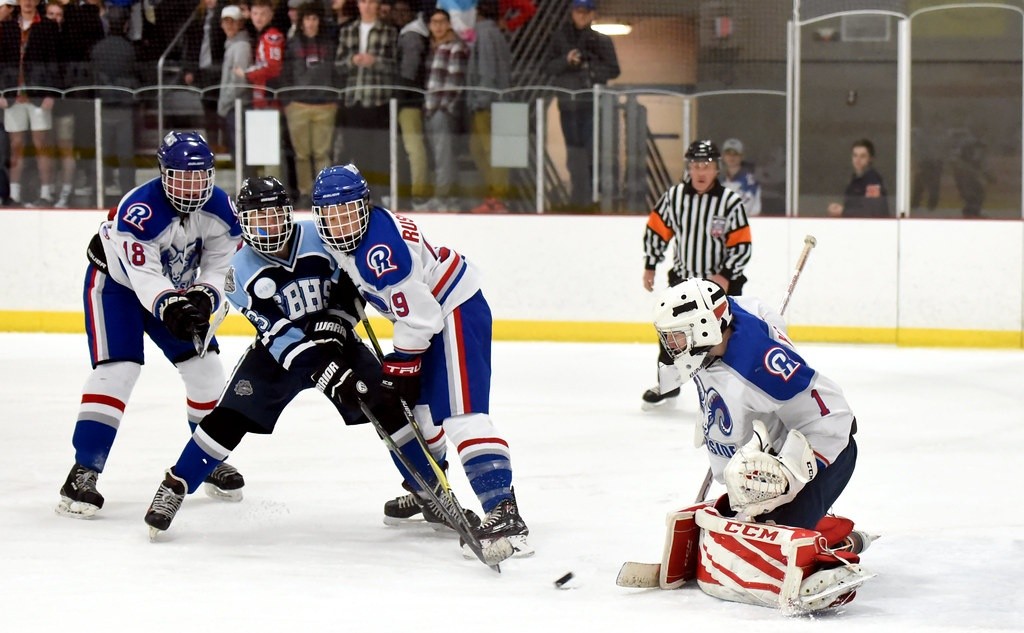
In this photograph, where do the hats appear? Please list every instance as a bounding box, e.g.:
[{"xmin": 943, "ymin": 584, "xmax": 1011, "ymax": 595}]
[
  {"xmin": 221, "ymin": 5, "xmax": 242, "ymax": 21},
  {"xmin": 723, "ymin": 138, "xmax": 743, "ymax": 153},
  {"xmin": 572, "ymin": 0, "xmax": 597, "ymax": 11}
]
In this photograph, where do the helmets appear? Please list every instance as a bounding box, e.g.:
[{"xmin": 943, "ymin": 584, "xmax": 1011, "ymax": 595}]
[
  {"xmin": 655, "ymin": 277, "xmax": 731, "ymax": 386},
  {"xmin": 312, "ymin": 164, "xmax": 370, "ymax": 253},
  {"xmin": 685, "ymin": 140, "xmax": 721, "ymax": 163},
  {"xmin": 235, "ymin": 177, "xmax": 294, "ymax": 254},
  {"xmin": 158, "ymin": 130, "xmax": 215, "ymax": 213}
]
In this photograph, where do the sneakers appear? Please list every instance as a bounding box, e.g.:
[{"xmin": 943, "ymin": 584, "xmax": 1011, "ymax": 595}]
[
  {"xmin": 641, "ymin": 382, "xmax": 680, "ymax": 412},
  {"xmin": 56, "ymin": 460, "xmax": 104, "ymax": 519},
  {"xmin": 202, "ymin": 461, "xmax": 244, "ymax": 502},
  {"xmin": 462, "ymin": 487, "xmax": 535, "ymax": 560},
  {"xmin": 383, "ymin": 460, "xmax": 449, "ymax": 527},
  {"xmin": 401, "ymin": 464, "xmax": 480, "ymax": 535},
  {"xmin": 836, "ymin": 529, "xmax": 883, "ymax": 555},
  {"xmin": 145, "ymin": 467, "xmax": 189, "ymax": 540}
]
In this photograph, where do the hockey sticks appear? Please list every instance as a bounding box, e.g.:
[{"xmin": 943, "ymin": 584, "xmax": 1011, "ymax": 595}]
[
  {"xmin": 192, "ymin": 301, "xmax": 230, "ymax": 360},
  {"xmin": 354, "ymin": 297, "xmax": 502, "ymax": 575},
  {"xmin": 615, "ymin": 234, "xmax": 819, "ymax": 589},
  {"xmin": 358, "ymin": 401, "xmax": 515, "ymax": 566}
]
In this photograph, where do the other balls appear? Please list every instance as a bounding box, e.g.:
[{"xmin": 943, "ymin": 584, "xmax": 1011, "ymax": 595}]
[{"xmin": 554, "ymin": 573, "xmax": 574, "ymax": 588}]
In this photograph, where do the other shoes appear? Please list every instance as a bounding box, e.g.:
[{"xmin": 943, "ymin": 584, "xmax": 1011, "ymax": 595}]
[
  {"xmin": 415, "ymin": 194, "xmax": 460, "ymax": 212},
  {"xmin": 105, "ymin": 184, "xmax": 123, "ymax": 196},
  {"xmin": 54, "ymin": 198, "xmax": 69, "ymax": 209},
  {"xmin": 24, "ymin": 197, "xmax": 55, "ymax": 209},
  {"xmin": 470, "ymin": 196, "xmax": 509, "ymax": 214},
  {"xmin": 0, "ymin": 197, "xmax": 22, "ymax": 208},
  {"xmin": 75, "ymin": 184, "xmax": 94, "ymax": 196}
]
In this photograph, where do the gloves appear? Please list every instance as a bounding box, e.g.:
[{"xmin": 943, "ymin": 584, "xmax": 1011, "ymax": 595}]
[
  {"xmin": 157, "ymin": 292, "xmax": 210, "ymax": 341},
  {"xmin": 315, "ymin": 314, "xmax": 346, "ymax": 357},
  {"xmin": 184, "ymin": 284, "xmax": 217, "ymax": 320},
  {"xmin": 332, "ymin": 268, "xmax": 367, "ymax": 322},
  {"xmin": 315, "ymin": 353, "xmax": 372, "ymax": 410},
  {"xmin": 380, "ymin": 352, "xmax": 423, "ymax": 411}
]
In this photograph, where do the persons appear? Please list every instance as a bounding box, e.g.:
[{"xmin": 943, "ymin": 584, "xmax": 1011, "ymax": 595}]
[
  {"xmin": 144, "ymin": 176, "xmax": 482, "ymax": 542},
  {"xmin": 827, "ymin": 138, "xmax": 890, "ymax": 218},
  {"xmin": 652, "ymin": 276, "xmax": 882, "ymax": 614},
  {"xmin": 720, "ymin": 139, "xmax": 761, "ymax": 217},
  {"xmin": 912, "ymin": 133, "xmax": 997, "ymax": 216},
  {"xmin": 54, "ymin": 131, "xmax": 244, "ymax": 518},
  {"xmin": 0, "ymin": 0, "xmax": 621, "ymax": 213},
  {"xmin": 312, "ymin": 163, "xmax": 545, "ymax": 558},
  {"xmin": 642, "ymin": 139, "xmax": 751, "ymax": 411}
]
